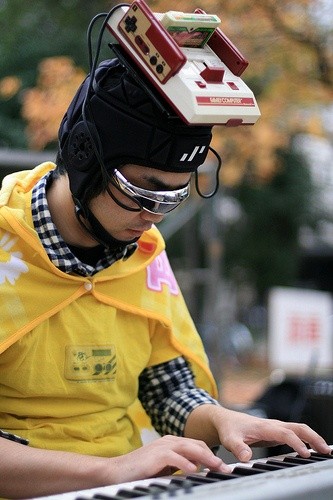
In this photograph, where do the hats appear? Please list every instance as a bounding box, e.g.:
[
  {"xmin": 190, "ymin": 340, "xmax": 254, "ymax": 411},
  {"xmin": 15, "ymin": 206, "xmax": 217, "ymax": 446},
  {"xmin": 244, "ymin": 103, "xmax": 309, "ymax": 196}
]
[{"xmin": 59, "ymin": 58, "xmax": 212, "ymax": 203}]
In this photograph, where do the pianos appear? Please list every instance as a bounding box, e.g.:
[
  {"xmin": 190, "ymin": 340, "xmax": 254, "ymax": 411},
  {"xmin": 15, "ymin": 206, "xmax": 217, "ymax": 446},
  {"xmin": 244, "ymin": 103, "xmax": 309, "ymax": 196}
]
[{"xmin": 26, "ymin": 444, "xmax": 333, "ymax": 500}]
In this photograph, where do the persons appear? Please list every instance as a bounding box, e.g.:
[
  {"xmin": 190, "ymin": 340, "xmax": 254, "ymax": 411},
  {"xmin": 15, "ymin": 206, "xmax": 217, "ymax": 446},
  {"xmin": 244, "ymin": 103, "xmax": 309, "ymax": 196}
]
[{"xmin": 0, "ymin": 52, "xmax": 332, "ymax": 495}]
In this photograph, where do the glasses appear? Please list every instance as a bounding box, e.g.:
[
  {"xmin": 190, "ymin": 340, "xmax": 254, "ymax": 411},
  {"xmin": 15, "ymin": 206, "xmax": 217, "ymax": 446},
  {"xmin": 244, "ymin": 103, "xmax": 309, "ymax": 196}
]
[{"xmin": 112, "ymin": 168, "xmax": 191, "ymax": 215}]
